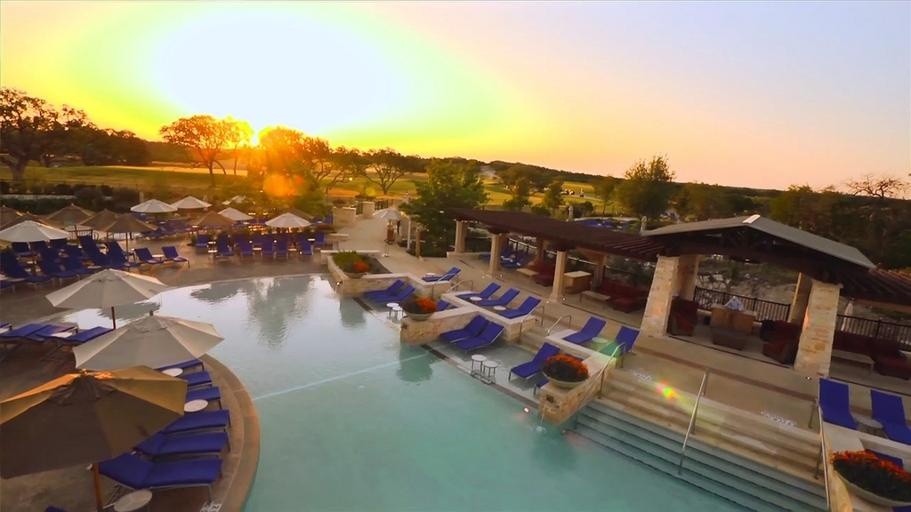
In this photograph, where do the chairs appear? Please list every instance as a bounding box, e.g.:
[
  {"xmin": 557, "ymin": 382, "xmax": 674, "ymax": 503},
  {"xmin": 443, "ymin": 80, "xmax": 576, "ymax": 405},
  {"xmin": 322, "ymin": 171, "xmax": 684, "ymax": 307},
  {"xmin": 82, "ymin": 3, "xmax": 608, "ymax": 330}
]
[
  {"xmin": 817, "ymin": 375, "xmax": 856, "ymax": 430},
  {"xmin": 476, "ymin": 246, "xmax": 646, "ymax": 313},
  {"xmin": 423, "ymin": 266, "xmax": 461, "ymax": 283},
  {"xmin": 0, "ymin": 214, "xmax": 334, "ymax": 293},
  {"xmin": 871, "ymin": 388, "xmax": 911, "ymax": 446},
  {"xmin": 458, "ymin": 282, "xmax": 499, "ymax": 300},
  {"xmin": 598, "ymin": 326, "xmax": 639, "ymax": 358},
  {"xmin": 366, "ymin": 280, "xmax": 416, "ymax": 305},
  {"xmin": 667, "ymin": 294, "xmax": 910, "ymax": 381},
  {"xmin": 565, "ymin": 317, "xmax": 606, "ymax": 344},
  {"xmin": 500, "ymin": 296, "xmax": 541, "ymax": 319},
  {"xmin": 507, "ymin": 343, "xmax": 560, "ymax": 387},
  {"xmin": 0, "ymin": 322, "xmax": 231, "ymax": 512},
  {"xmin": 439, "ymin": 315, "xmax": 490, "ymax": 344},
  {"xmin": 436, "ymin": 300, "xmax": 448, "ymax": 313},
  {"xmin": 455, "ymin": 321, "xmax": 505, "ymax": 359}
]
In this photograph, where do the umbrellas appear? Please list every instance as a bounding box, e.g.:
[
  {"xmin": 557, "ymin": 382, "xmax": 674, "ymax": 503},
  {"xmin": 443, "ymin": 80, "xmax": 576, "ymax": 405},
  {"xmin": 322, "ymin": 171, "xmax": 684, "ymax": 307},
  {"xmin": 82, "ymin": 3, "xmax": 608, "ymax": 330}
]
[
  {"xmin": 70, "ymin": 310, "xmax": 223, "ymax": 372},
  {"xmin": 370, "ymin": 205, "xmax": 407, "ymax": 226},
  {"xmin": 1, "ymin": 364, "xmax": 189, "ymax": 511},
  {"xmin": 44, "ymin": 265, "xmax": 167, "ymax": 329}
]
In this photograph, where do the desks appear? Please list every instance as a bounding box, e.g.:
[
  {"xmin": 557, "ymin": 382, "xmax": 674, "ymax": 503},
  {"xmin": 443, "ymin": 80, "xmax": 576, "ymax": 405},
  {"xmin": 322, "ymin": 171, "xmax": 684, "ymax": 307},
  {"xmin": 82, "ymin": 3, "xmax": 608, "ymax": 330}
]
[
  {"xmin": 392, "ymin": 307, "xmax": 404, "ymax": 321},
  {"xmin": 386, "ymin": 302, "xmax": 399, "ymax": 320},
  {"xmin": 470, "ymin": 354, "xmax": 486, "ymax": 376},
  {"xmin": 849, "ymin": 412, "xmax": 883, "ymax": 435},
  {"xmin": 481, "ymin": 361, "xmax": 498, "ymax": 378}
]
